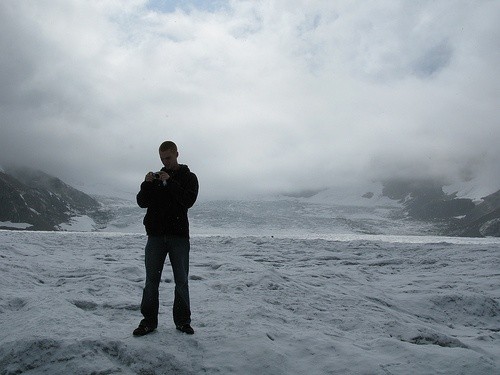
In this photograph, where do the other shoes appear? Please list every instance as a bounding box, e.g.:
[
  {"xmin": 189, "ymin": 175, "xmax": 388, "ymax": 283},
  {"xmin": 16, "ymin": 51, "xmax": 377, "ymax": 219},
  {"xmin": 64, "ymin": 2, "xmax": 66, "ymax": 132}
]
[
  {"xmin": 175, "ymin": 323, "xmax": 194, "ymax": 334},
  {"xmin": 132, "ymin": 319, "xmax": 157, "ymax": 335}
]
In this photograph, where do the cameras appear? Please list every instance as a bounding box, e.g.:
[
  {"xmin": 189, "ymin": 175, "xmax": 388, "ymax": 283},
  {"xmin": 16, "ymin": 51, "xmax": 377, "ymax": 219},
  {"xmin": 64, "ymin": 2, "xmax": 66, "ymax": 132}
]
[{"xmin": 149, "ymin": 172, "xmax": 162, "ymax": 180}]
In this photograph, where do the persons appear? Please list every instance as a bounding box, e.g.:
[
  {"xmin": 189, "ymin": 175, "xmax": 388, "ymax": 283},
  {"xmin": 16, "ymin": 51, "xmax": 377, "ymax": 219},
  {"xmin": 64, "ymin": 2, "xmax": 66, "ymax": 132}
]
[{"xmin": 131, "ymin": 140, "xmax": 199, "ymax": 338}]
